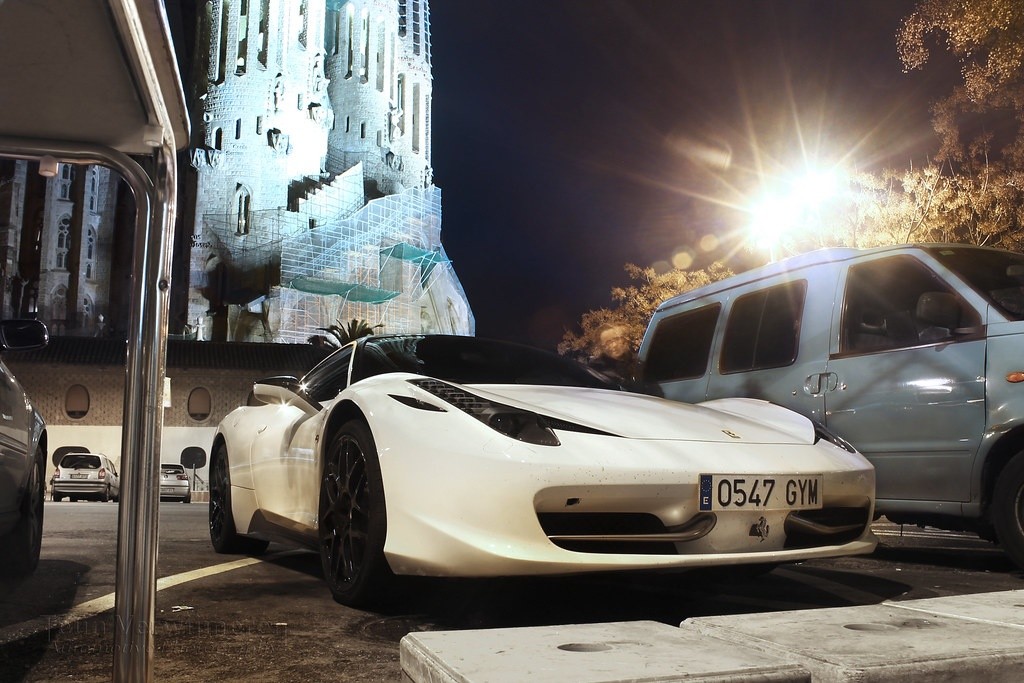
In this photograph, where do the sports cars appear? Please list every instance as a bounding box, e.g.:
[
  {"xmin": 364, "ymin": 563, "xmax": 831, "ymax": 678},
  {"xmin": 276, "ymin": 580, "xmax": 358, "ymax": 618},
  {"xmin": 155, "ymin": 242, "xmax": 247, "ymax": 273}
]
[{"xmin": 207, "ymin": 331, "xmax": 879, "ymax": 613}]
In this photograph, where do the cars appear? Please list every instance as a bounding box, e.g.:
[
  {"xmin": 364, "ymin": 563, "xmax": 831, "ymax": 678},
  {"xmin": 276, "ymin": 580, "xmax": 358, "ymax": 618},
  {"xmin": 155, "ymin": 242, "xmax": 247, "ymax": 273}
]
[
  {"xmin": 160, "ymin": 462, "xmax": 191, "ymax": 503},
  {"xmin": 0, "ymin": 313, "xmax": 54, "ymax": 601}
]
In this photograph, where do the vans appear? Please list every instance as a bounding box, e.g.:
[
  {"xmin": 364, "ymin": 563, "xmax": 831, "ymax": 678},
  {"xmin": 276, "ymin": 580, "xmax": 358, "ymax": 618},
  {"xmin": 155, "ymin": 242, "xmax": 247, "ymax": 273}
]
[{"xmin": 637, "ymin": 243, "xmax": 1024, "ymax": 569}]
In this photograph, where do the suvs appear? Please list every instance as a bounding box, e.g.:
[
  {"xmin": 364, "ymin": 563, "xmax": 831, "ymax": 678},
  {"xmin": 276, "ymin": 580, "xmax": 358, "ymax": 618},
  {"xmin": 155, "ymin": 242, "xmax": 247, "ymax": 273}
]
[{"xmin": 51, "ymin": 453, "xmax": 120, "ymax": 503}]
